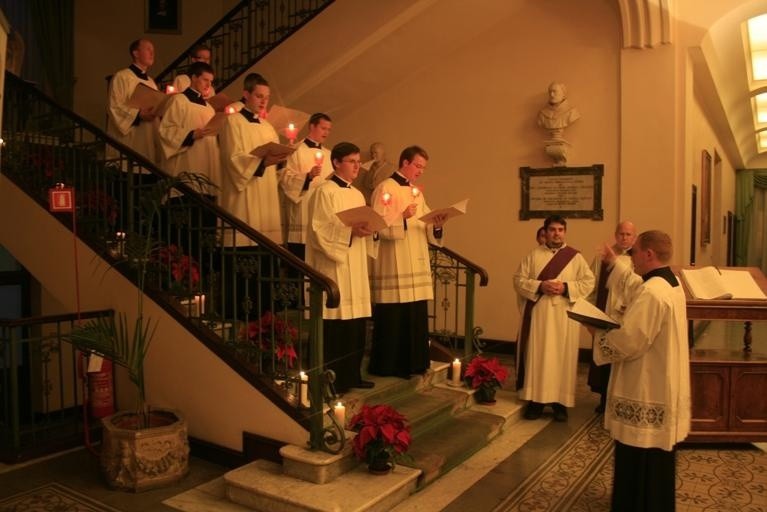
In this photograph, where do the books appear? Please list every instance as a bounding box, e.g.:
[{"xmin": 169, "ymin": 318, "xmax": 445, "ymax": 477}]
[
  {"xmin": 417, "ymin": 197, "xmax": 469, "ymax": 225},
  {"xmin": 678, "ymin": 266, "xmax": 766, "ymax": 304},
  {"xmin": 202, "ymin": 108, "xmax": 229, "ymax": 140},
  {"xmin": 336, "ymin": 204, "xmax": 404, "ymax": 236},
  {"xmin": 127, "ymin": 83, "xmax": 178, "ymax": 121},
  {"xmin": 249, "ymin": 139, "xmax": 309, "ymax": 161},
  {"xmin": 566, "ymin": 294, "xmax": 621, "ymax": 333}
]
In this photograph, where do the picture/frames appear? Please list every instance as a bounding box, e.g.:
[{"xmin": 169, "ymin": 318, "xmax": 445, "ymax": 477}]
[{"xmin": 517, "ymin": 164, "xmax": 604, "ymax": 221}]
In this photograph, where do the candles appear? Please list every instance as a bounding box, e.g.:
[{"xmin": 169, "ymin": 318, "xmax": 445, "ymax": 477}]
[{"xmin": 165, "ymin": 83, "xmax": 419, "ymax": 207}]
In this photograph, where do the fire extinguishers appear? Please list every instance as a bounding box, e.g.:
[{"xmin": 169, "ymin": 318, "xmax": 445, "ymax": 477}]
[{"xmin": 88, "ymin": 350, "xmax": 115, "ymax": 418}]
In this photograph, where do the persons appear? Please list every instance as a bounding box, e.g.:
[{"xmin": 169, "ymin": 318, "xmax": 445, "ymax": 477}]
[
  {"xmin": 301, "ymin": 141, "xmax": 375, "ymax": 396},
  {"xmin": 102, "ymin": 38, "xmax": 163, "ymax": 236},
  {"xmin": 154, "ymin": 61, "xmax": 220, "ymax": 249},
  {"xmin": 364, "ymin": 144, "xmax": 451, "ymax": 379},
  {"xmin": 535, "ymin": 83, "xmax": 580, "ymax": 133},
  {"xmin": 223, "ymin": 73, "xmax": 260, "ymax": 122},
  {"xmin": 281, "ymin": 112, "xmax": 335, "ymax": 308},
  {"xmin": 580, "ymin": 229, "xmax": 692, "ymax": 510},
  {"xmin": 361, "ymin": 142, "xmax": 395, "ymax": 191},
  {"xmin": 172, "ymin": 46, "xmax": 215, "ymax": 102},
  {"xmin": 586, "ymin": 221, "xmax": 640, "ymax": 413},
  {"xmin": 514, "ymin": 228, "xmax": 547, "ymax": 312},
  {"xmin": 511, "ymin": 214, "xmax": 596, "ymax": 423},
  {"xmin": 216, "ymin": 76, "xmax": 297, "ymax": 324}
]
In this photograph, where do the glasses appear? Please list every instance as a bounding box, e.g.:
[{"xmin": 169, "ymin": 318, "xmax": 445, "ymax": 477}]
[{"xmin": 344, "ymin": 158, "xmax": 361, "ymax": 164}]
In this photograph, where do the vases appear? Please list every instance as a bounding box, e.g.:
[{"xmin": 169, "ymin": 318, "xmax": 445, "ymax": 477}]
[{"xmin": 368, "ymin": 451, "xmax": 391, "ymax": 474}]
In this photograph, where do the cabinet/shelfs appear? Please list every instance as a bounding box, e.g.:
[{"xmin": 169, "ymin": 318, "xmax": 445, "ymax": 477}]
[{"xmin": 683, "ymin": 349, "xmax": 767, "ymax": 444}]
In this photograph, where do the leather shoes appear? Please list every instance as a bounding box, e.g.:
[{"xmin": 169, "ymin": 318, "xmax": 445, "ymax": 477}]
[{"xmin": 350, "ymin": 379, "xmax": 374, "ymax": 389}]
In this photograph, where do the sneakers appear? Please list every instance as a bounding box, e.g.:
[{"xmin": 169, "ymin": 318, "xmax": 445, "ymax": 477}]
[
  {"xmin": 553, "ymin": 409, "xmax": 569, "ymax": 421},
  {"xmin": 525, "ymin": 405, "xmax": 543, "ymax": 419}
]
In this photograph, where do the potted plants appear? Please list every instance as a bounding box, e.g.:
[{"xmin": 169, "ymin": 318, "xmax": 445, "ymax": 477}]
[{"xmin": 53, "ymin": 154, "xmax": 222, "ymax": 496}]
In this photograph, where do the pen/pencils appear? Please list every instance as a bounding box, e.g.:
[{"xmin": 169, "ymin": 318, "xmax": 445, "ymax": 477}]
[{"xmin": 713, "ymin": 264, "xmax": 722, "ymax": 275}]
